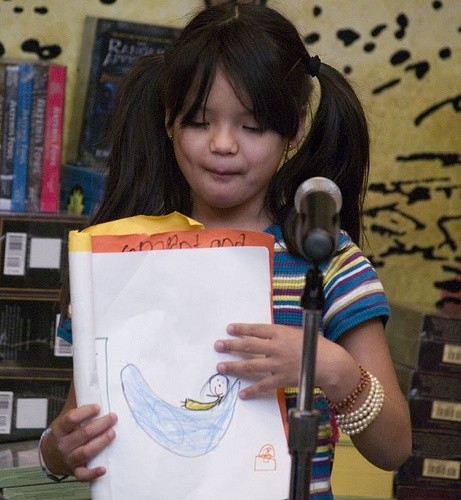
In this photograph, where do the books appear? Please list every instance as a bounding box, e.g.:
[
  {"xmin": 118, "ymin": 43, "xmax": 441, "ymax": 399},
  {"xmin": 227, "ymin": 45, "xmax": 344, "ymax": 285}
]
[
  {"xmin": 0, "ymin": 304, "xmax": 73, "ymax": 441},
  {"xmin": 0, "ymin": 16, "xmax": 187, "ymax": 215}
]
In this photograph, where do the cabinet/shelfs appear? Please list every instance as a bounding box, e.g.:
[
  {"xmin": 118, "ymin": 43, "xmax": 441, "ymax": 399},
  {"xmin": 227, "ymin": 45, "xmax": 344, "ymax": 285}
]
[{"xmin": 0, "ymin": 214, "xmax": 89, "ymax": 442}]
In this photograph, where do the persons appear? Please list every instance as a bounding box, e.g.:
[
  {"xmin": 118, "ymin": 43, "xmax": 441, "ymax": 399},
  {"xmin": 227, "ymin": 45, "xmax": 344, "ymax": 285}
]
[{"xmin": 37, "ymin": 2, "xmax": 411, "ymax": 500}]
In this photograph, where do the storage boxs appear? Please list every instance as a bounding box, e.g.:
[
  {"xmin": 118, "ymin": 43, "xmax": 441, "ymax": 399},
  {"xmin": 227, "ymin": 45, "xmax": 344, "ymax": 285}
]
[{"xmin": 0, "ymin": 467, "xmax": 91, "ymax": 498}]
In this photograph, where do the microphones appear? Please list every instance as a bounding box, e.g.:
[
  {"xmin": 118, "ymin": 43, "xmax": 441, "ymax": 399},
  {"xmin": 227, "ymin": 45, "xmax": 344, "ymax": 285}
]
[{"xmin": 294, "ymin": 176, "xmax": 343, "ymax": 264}]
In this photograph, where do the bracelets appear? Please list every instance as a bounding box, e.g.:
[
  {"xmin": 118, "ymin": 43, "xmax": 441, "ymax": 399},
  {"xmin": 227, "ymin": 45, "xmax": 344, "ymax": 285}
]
[
  {"xmin": 334, "ymin": 372, "xmax": 385, "ymax": 435},
  {"xmin": 325, "ymin": 366, "xmax": 368, "ymax": 442},
  {"xmin": 37, "ymin": 428, "xmax": 68, "ymax": 481}
]
